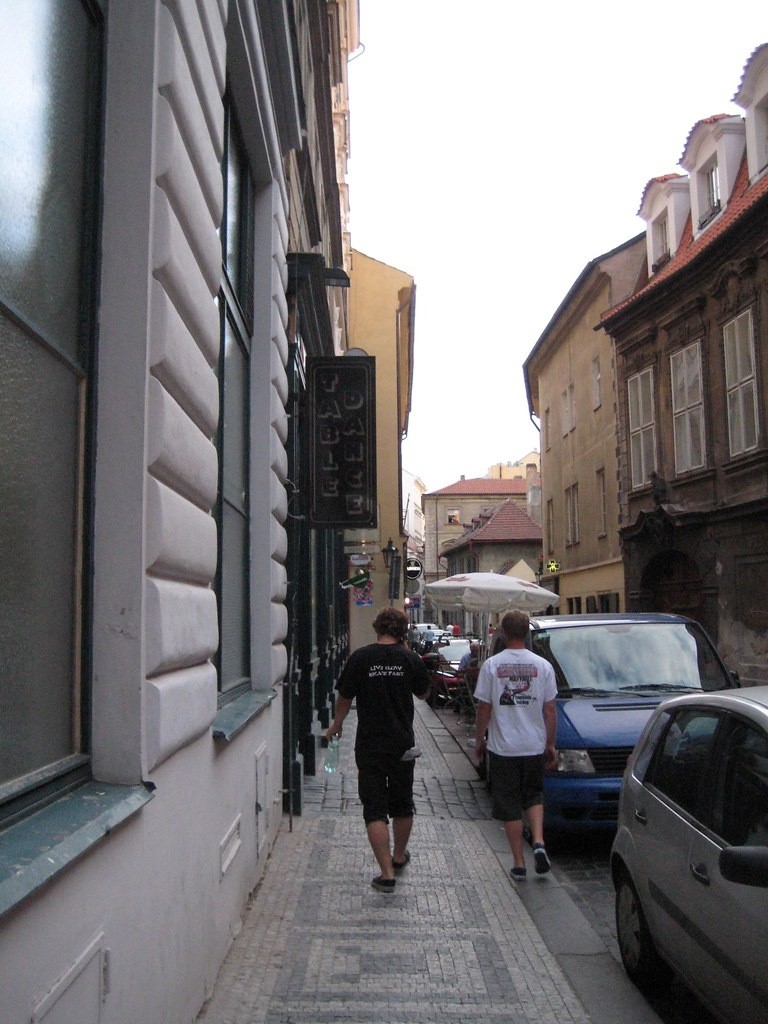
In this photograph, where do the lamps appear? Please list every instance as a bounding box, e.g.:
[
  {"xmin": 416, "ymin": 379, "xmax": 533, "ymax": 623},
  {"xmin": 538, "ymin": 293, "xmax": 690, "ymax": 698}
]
[
  {"xmin": 323, "ymin": 266, "xmax": 353, "ymax": 289},
  {"xmin": 379, "ymin": 536, "xmax": 398, "ymax": 570}
]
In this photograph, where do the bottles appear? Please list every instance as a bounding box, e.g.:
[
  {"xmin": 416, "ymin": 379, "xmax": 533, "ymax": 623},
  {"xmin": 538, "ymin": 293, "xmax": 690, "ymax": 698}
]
[{"xmin": 324, "ymin": 732, "xmax": 340, "ymax": 774}]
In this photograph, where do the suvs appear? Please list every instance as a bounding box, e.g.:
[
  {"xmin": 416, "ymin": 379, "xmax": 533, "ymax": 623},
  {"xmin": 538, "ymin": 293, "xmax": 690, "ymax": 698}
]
[{"xmin": 481, "ymin": 611, "xmax": 745, "ymax": 854}]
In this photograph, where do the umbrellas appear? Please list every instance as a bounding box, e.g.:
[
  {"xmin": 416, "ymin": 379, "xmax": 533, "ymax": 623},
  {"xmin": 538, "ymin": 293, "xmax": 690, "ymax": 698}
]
[{"xmin": 423, "ymin": 567, "xmax": 559, "ymax": 665}]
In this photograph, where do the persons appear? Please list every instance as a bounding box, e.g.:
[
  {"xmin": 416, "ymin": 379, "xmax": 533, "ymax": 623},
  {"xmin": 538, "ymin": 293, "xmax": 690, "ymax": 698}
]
[
  {"xmin": 423, "ymin": 626, "xmax": 434, "ymax": 649},
  {"xmin": 454, "ymin": 643, "xmax": 490, "ymax": 715},
  {"xmin": 326, "ymin": 608, "xmax": 431, "ymax": 892},
  {"xmin": 473, "ymin": 611, "xmax": 559, "ymax": 879},
  {"xmin": 410, "ymin": 621, "xmax": 416, "ymax": 629},
  {"xmin": 488, "ymin": 624, "xmax": 494, "ymax": 637},
  {"xmin": 453, "ymin": 623, "xmax": 461, "ymax": 637},
  {"xmin": 409, "ymin": 626, "xmax": 422, "ymax": 653},
  {"xmin": 446, "ymin": 623, "xmax": 454, "ymax": 636}
]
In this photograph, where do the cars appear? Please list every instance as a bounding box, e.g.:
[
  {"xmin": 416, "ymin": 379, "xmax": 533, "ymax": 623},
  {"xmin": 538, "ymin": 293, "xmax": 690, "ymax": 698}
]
[
  {"xmin": 605, "ymin": 684, "xmax": 768, "ymax": 1024},
  {"xmin": 408, "ymin": 622, "xmax": 483, "ymax": 702}
]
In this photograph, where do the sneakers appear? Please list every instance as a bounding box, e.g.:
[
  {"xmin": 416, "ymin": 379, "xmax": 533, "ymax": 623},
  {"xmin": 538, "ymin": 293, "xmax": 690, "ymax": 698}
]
[
  {"xmin": 533, "ymin": 842, "xmax": 552, "ymax": 875},
  {"xmin": 509, "ymin": 867, "xmax": 527, "ymax": 881},
  {"xmin": 392, "ymin": 849, "xmax": 412, "ymax": 873},
  {"xmin": 371, "ymin": 875, "xmax": 396, "ymax": 893}
]
